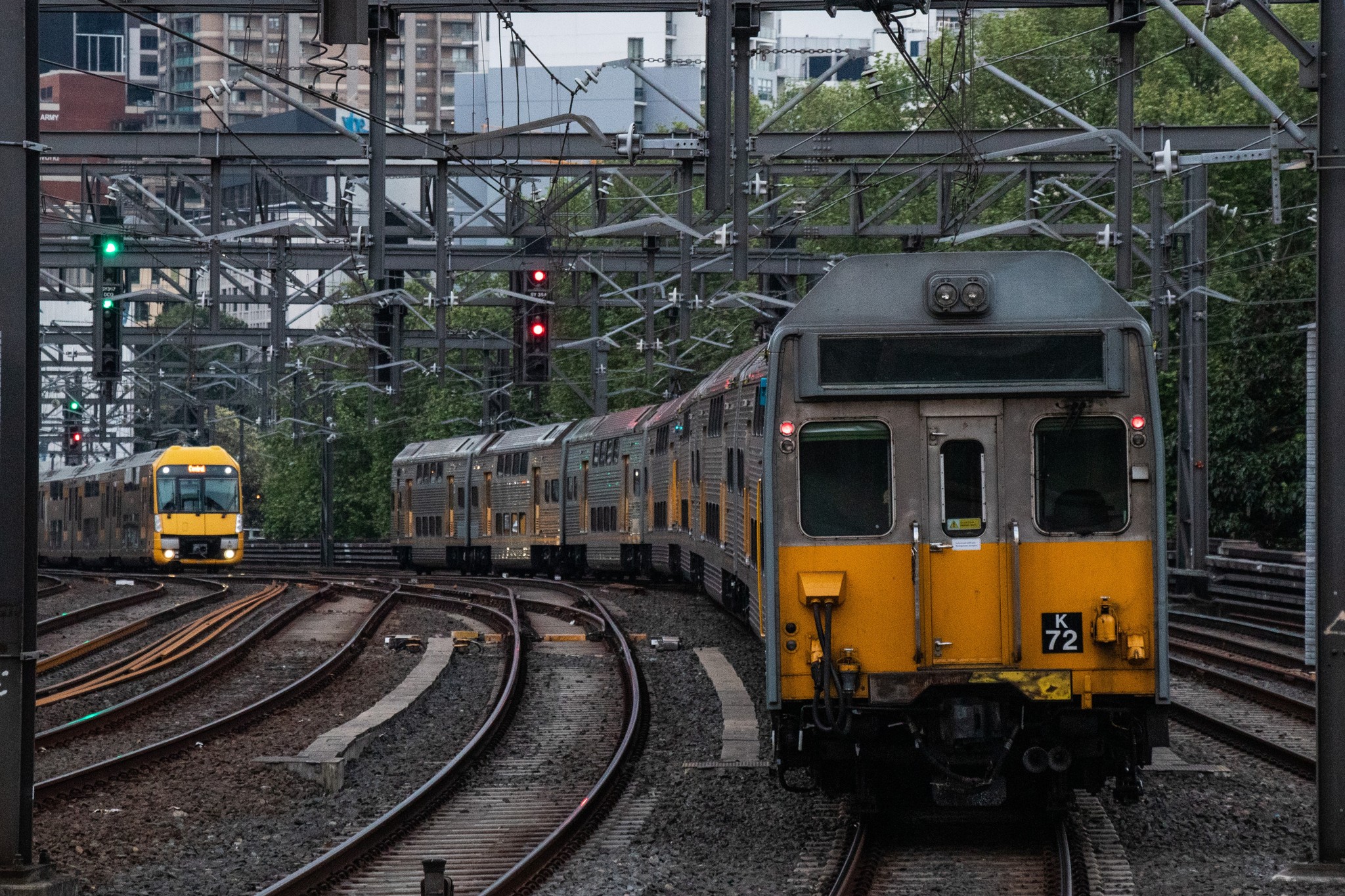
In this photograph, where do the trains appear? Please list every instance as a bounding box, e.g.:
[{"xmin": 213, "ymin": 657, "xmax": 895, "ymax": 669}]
[
  {"xmin": 393, "ymin": 249, "xmax": 1170, "ymax": 848},
  {"xmin": 37, "ymin": 446, "xmax": 244, "ymax": 575}
]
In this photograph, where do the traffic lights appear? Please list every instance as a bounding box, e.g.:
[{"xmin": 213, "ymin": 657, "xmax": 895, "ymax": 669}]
[
  {"xmin": 372, "ymin": 213, "xmax": 408, "ymax": 382},
  {"xmin": 93, "ymin": 206, "xmax": 122, "ymax": 384},
  {"xmin": 62, "ymin": 390, "xmax": 84, "ymax": 466},
  {"xmin": 510, "ymin": 243, "xmax": 552, "ymax": 387}
]
